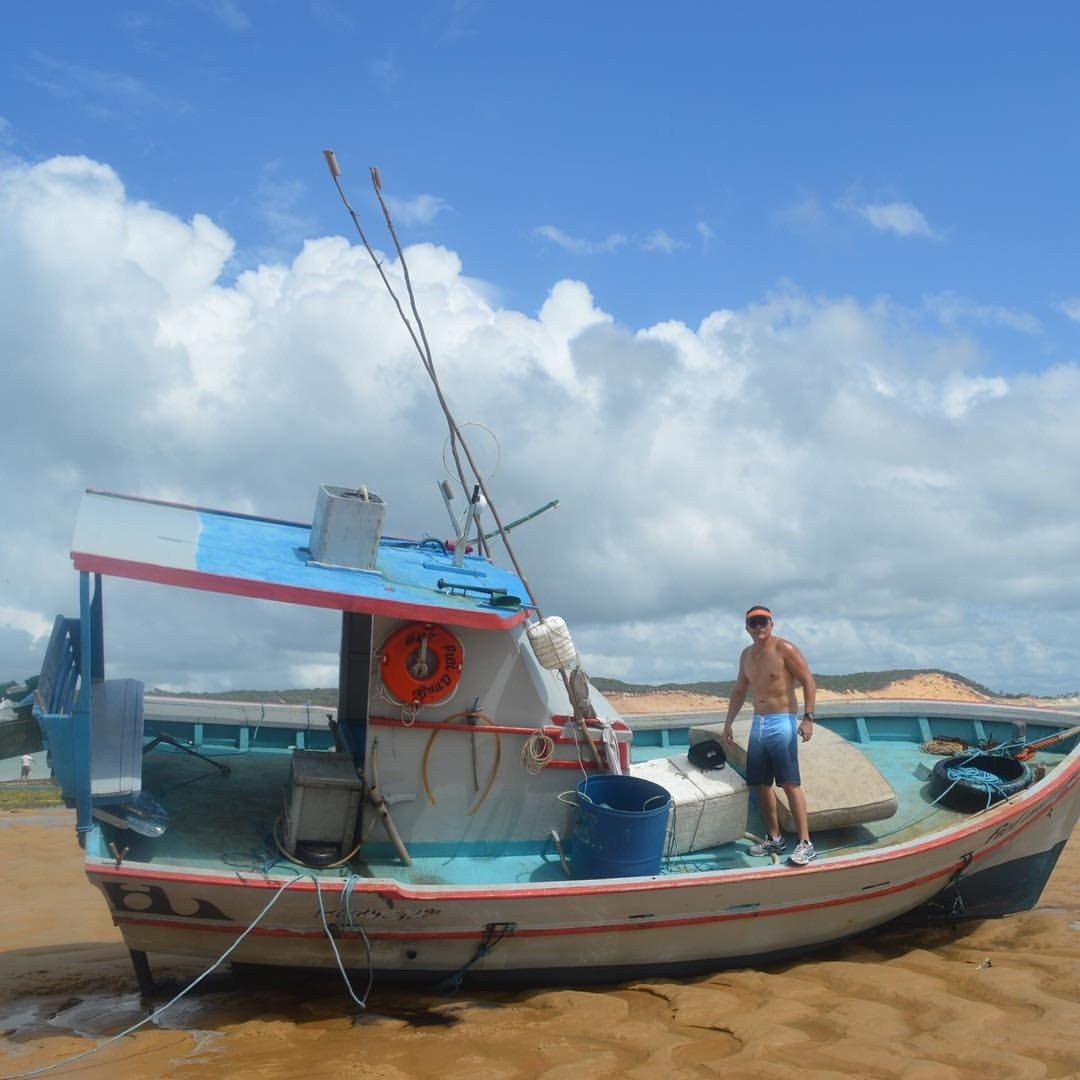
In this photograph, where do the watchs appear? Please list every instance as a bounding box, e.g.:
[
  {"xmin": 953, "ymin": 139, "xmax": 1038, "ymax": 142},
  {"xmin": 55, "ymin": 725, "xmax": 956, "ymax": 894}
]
[{"xmin": 803, "ymin": 711, "xmax": 815, "ymax": 721}]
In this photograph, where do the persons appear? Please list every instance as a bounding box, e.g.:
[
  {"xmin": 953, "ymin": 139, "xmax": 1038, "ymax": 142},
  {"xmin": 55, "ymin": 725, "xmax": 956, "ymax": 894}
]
[
  {"xmin": 20, "ymin": 754, "xmax": 34, "ymax": 784},
  {"xmin": 724, "ymin": 606, "xmax": 817, "ymax": 866}
]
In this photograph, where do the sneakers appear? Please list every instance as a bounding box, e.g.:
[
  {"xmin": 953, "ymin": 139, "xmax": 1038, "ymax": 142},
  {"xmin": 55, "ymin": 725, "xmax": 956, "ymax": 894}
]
[
  {"xmin": 749, "ymin": 834, "xmax": 785, "ymax": 857},
  {"xmin": 788, "ymin": 840, "xmax": 816, "ymax": 865}
]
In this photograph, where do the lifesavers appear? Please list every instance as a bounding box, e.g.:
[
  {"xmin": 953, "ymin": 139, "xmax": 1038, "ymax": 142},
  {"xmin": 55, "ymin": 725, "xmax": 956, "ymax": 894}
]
[
  {"xmin": 930, "ymin": 753, "xmax": 1033, "ymax": 813},
  {"xmin": 378, "ymin": 623, "xmax": 464, "ymax": 704}
]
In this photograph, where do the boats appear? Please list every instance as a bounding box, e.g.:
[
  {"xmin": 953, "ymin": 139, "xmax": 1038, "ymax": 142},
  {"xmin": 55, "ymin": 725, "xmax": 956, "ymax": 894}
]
[{"xmin": 26, "ymin": 145, "xmax": 1072, "ymax": 989}]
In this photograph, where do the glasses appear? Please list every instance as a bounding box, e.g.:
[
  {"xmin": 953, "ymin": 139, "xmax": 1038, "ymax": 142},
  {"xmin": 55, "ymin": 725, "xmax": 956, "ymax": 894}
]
[{"xmin": 747, "ymin": 618, "xmax": 772, "ymax": 629}]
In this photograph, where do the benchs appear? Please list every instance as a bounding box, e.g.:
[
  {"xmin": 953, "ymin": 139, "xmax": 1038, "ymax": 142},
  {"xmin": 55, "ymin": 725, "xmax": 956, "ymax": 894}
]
[{"xmin": 281, "ymin": 747, "xmax": 361, "ymax": 859}]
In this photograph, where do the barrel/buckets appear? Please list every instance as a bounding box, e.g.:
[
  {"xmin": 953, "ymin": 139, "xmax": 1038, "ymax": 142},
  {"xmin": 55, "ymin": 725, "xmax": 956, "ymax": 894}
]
[{"xmin": 570, "ymin": 773, "xmax": 671, "ymax": 879}]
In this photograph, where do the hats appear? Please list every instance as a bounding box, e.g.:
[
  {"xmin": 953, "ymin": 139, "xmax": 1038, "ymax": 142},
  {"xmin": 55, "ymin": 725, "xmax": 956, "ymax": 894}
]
[{"xmin": 745, "ymin": 609, "xmax": 772, "ymax": 623}]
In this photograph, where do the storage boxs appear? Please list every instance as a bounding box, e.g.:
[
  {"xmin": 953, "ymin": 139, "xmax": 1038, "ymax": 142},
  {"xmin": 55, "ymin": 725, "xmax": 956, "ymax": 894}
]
[{"xmin": 628, "ymin": 752, "xmax": 751, "ymax": 856}]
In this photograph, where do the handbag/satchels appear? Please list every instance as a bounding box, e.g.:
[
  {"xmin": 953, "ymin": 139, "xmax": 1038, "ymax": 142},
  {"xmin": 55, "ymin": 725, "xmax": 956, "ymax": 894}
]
[{"xmin": 688, "ymin": 740, "xmax": 726, "ymax": 771}]
